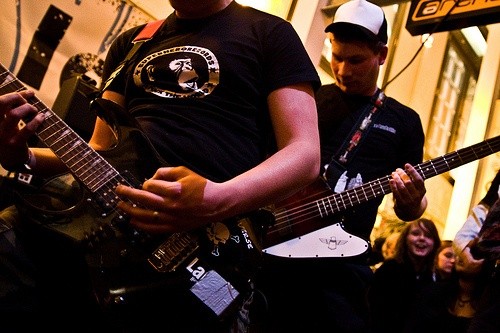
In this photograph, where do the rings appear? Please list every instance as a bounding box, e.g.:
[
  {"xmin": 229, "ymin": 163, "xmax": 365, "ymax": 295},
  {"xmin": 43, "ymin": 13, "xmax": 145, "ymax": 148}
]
[{"xmin": 152, "ymin": 211, "xmax": 161, "ymax": 223}]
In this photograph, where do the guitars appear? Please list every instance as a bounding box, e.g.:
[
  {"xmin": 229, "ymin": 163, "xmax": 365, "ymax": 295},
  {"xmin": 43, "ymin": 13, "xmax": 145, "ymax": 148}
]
[
  {"xmin": 0, "ymin": 65, "xmax": 268, "ymax": 333},
  {"xmin": 96, "ymin": 135, "xmax": 500, "ymax": 333}
]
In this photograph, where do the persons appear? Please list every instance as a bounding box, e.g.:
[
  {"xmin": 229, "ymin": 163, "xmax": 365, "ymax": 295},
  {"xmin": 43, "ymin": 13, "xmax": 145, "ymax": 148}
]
[
  {"xmin": 0, "ymin": 0, "xmax": 500, "ymax": 333},
  {"xmin": 260, "ymin": 0, "xmax": 428, "ymax": 333}
]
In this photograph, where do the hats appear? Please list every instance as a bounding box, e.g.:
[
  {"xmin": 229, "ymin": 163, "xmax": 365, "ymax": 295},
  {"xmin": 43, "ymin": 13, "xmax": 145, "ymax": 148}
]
[{"xmin": 324, "ymin": 1, "xmax": 388, "ymax": 43}]
